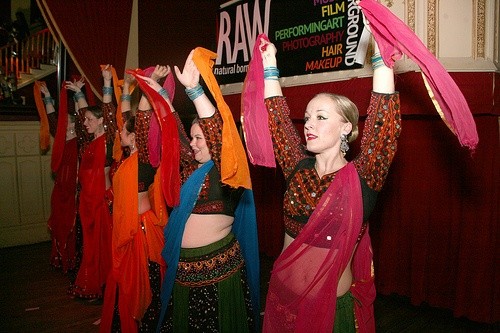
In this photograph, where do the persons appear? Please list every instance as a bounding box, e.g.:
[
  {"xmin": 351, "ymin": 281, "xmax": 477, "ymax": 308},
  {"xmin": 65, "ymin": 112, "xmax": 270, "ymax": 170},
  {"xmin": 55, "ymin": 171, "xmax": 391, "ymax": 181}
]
[
  {"xmin": 65, "ymin": 65, "xmax": 123, "ymax": 303},
  {"xmin": 259, "ymin": 0, "xmax": 402, "ymax": 333},
  {"xmin": 129, "ymin": 47, "xmax": 257, "ymax": 333},
  {"xmin": 99, "ymin": 65, "xmax": 170, "ymax": 333},
  {"xmin": 36, "ymin": 77, "xmax": 85, "ymax": 272},
  {"xmin": 11, "ymin": 12, "xmax": 31, "ymax": 69}
]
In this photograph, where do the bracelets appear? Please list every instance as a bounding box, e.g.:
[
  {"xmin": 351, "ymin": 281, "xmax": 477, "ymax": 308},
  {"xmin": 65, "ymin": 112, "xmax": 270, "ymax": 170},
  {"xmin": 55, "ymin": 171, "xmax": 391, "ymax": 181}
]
[
  {"xmin": 158, "ymin": 88, "xmax": 169, "ymax": 98},
  {"xmin": 185, "ymin": 84, "xmax": 204, "ymax": 100},
  {"xmin": 103, "ymin": 87, "xmax": 112, "ymax": 94},
  {"xmin": 75, "ymin": 92, "xmax": 84, "ymax": 99},
  {"xmin": 264, "ymin": 67, "xmax": 279, "ymax": 80},
  {"xmin": 120, "ymin": 94, "xmax": 130, "ymax": 101},
  {"xmin": 371, "ymin": 52, "xmax": 385, "ymax": 69},
  {"xmin": 73, "ymin": 96, "xmax": 77, "ymax": 101},
  {"xmin": 44, "ymin": 96, "xmax": 51, "ymax": 104}
]
